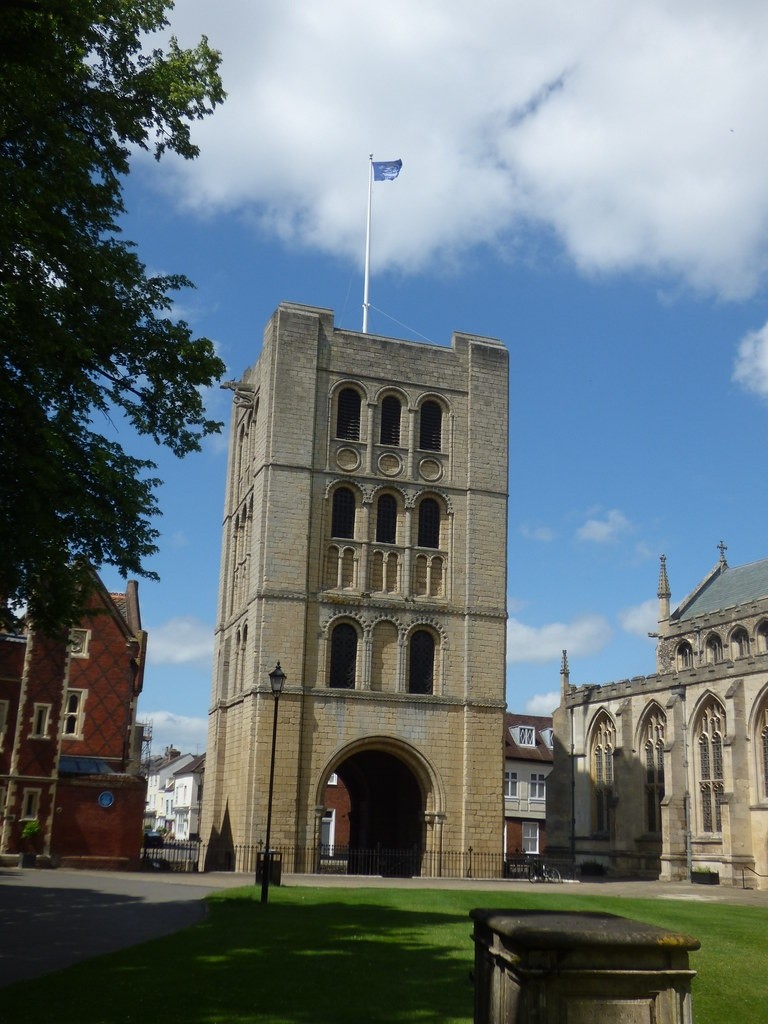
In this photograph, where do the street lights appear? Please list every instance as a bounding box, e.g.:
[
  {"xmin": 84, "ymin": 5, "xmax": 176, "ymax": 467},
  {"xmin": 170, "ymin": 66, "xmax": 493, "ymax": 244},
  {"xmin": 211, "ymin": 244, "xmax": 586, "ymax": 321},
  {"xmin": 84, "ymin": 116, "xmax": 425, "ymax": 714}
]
[{"xmin": 258, "ymin": 658, "xmax": 287, "ymax": 903}]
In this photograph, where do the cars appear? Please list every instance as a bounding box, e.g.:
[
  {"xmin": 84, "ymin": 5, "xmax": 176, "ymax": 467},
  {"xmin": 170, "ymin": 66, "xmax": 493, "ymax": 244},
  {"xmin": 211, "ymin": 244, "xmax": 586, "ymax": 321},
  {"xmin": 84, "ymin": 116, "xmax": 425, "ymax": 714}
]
[{"xmin": 144, "ymin": 832, "xmax": 164, "ymax": 848}]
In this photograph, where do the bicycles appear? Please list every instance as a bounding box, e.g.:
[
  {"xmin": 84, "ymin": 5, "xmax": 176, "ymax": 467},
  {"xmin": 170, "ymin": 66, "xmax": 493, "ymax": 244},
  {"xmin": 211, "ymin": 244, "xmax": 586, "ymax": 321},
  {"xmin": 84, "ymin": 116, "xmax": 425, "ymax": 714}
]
[{"xmin": 526, "ymin": 858, "xmax": 562, "ymax": 886}]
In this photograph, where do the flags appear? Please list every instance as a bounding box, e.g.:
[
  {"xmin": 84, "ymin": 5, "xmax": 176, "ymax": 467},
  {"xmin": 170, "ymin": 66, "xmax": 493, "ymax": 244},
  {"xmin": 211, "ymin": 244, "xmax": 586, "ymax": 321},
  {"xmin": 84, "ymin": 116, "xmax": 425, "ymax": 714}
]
[{"xmin": 372, "ymin": 159, "xmax": 403, "ymax": 182}]
